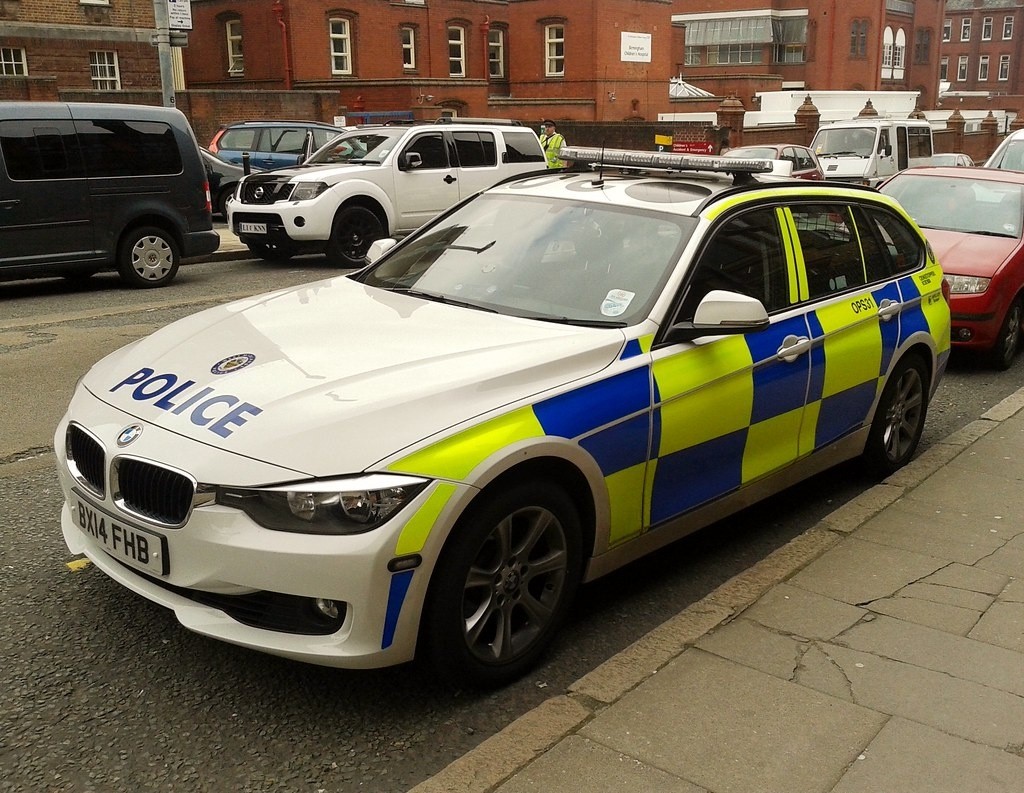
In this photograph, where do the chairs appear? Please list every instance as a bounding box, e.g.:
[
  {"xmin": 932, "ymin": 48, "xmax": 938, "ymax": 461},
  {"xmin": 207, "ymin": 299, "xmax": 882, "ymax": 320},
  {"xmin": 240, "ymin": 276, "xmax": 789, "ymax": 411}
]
[
  {"xmin": 557, "ymin": 226, "xmax": 625, "ymax": 300},
  {"xmin": 999, "ymin": 192, "xmax": 1024, "ymax": 220},
  {"xmin": 693, "ymin": 233, "xmax": 781, "ymax": 309}
]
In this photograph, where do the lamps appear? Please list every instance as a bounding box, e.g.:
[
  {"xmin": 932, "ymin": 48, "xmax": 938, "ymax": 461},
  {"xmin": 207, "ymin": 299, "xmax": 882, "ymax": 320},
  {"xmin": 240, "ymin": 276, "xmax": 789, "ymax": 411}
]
[{"xmin": 426, "ymin": 95, "xmax": 435, "ymax": 102}]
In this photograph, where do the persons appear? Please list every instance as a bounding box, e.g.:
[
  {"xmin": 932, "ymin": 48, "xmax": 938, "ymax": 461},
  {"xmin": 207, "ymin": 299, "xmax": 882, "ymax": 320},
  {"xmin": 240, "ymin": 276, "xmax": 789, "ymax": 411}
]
[{"xmin": 540, "ymin": 120, "xmax": 568, "ymax": 169}]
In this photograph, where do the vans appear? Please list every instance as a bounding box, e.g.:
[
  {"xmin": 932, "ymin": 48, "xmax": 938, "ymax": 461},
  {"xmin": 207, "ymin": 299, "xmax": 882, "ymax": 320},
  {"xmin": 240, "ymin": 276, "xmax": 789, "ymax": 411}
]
[
  {"xmin": 0, "ymin": 99, "xmax": 220, "ymax": 292},
  {"xmin": 810, "ymin": 119, "xmax": 935, "ymax": 189}
]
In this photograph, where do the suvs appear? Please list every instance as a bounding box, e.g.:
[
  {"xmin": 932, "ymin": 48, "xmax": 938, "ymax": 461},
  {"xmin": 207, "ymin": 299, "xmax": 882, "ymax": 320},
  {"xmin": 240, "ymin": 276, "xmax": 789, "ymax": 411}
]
[
  {"xmin": 226, "ymin": 118, "xmax": 548, "ymax": 270},
  {"xmin": 198, "ymin": 119, "xmax": 369, "ymax": 222}
]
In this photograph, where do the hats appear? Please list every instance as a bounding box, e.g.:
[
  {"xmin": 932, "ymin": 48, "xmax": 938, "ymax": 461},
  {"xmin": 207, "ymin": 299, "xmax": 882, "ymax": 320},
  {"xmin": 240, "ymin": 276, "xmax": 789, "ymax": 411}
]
[{"xmin": 543, "ymin": 119, "xmax": 557, "ymax": 127}]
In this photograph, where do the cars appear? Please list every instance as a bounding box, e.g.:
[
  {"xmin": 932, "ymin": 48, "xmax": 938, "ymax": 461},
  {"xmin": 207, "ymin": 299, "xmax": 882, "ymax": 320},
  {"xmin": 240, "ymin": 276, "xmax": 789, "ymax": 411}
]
[
  {"xmin": 722, "ymin": 144, "xmax": 824, "ymax": 178},
  {"xmin": 981, "ymin": 130, "xmax": 1024, "ymax": 172},
  {"xmin": 53, "ymin": 146, "xmax": 954, "ymax": 704},
  {"xmin": 875, "ymin": 164, "xmax": 1024, "ymax": 372},
  {"xmin": 933, "ymin": 153, "xmax": 976, "ymax": 166}
]
